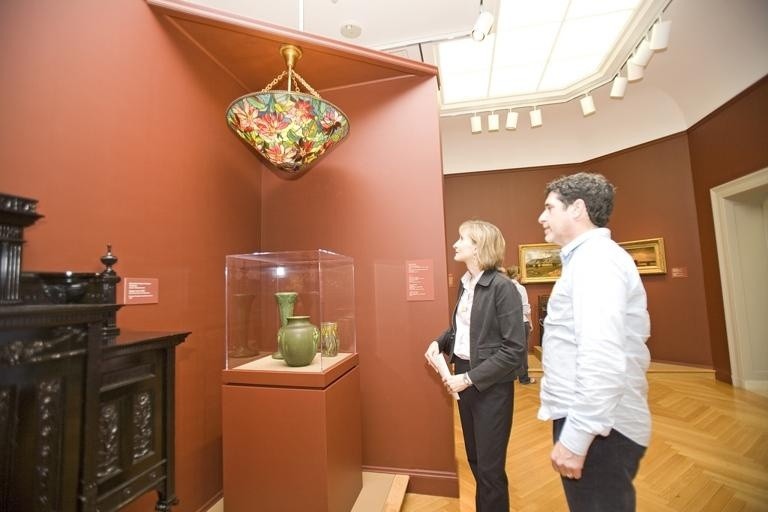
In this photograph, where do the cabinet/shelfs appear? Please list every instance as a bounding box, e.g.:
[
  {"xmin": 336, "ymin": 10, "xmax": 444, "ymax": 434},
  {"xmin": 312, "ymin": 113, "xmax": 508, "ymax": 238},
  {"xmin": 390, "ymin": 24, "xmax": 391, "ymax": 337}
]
[{"xmin": 0, "ymin": 192, "xmax": 192, "ymax": 512}]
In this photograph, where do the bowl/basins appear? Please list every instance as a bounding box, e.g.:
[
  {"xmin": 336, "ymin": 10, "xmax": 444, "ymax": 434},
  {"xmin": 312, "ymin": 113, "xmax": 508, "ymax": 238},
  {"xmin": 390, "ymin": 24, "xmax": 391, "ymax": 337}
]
[{"xmin": 36, "ymin": 271, "xmax": 95, "ymax": 303}]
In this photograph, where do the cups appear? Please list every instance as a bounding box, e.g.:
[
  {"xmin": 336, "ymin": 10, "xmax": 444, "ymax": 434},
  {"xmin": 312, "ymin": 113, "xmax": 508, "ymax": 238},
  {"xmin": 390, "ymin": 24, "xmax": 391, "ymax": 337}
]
[{"xmin": 319, "ymin": 321, "xmax": 339, "ymax": 356}]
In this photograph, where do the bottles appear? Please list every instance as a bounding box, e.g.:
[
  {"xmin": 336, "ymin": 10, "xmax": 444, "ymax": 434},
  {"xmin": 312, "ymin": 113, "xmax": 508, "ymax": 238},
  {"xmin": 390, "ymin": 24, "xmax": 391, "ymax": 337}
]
[
  {"xmin": 270, "ymin": 291, "xmax": 320, "ymax": 367},
  {"xmin": 229, "ymin": 293, "xmax": 261, "ymax": 359}
]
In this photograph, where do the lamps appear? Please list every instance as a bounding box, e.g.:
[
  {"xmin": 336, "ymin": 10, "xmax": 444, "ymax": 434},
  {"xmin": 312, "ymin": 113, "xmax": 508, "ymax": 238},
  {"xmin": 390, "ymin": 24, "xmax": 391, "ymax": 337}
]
[
  {"xmin": 472, "ymin": 0, "xmax": 494, "ymax": 42},
  {"xmin": 440, "ymin": 1, "xmax": 679, "ymax": 133},
  {"xmin": 224, "ymin": 45, "xmax": 350, "ymax": 175}
]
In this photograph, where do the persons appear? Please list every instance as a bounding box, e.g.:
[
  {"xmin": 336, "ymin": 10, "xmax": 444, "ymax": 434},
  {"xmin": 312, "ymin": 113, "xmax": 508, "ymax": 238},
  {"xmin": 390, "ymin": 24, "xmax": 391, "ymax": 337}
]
[
  {"xmin": 424, "ymin": 218, "xmax": 527, "ymax": 512},
  {"xmin": 535, "ymin": 172, "xmax": 652, "ymax": 512},
  {"xmin": 506, "ymin": 266, "xmax": 536, "ymax": 384}
]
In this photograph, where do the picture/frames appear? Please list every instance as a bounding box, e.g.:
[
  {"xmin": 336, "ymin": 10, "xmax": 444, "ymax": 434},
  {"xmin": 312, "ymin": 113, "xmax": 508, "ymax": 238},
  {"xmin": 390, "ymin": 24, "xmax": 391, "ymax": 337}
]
[
  {"xmin": 616, "ymin": 237, "xmax": 667, "ymax": 275},
  {"xmin": 518, "ymin": 242, "xmax": 562, "ymax": 285}
]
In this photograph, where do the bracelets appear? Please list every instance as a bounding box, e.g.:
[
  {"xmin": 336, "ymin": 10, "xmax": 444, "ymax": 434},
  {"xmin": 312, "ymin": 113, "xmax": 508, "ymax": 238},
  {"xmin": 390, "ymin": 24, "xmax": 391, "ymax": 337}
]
[{"xmin": 461, "ymin": 373, "xmax": 472, "ymax": 387}]
